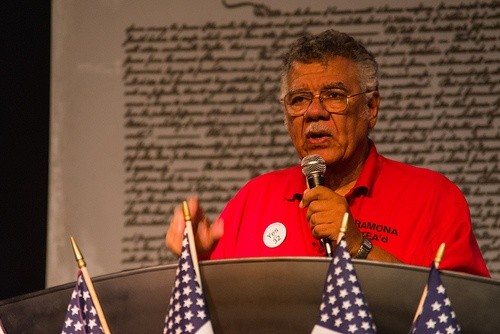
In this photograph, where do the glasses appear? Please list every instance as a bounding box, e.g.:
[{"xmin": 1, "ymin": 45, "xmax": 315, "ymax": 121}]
[{"xmin": 279, "ymin": 86, "xmax": 373, "ymax": 117}]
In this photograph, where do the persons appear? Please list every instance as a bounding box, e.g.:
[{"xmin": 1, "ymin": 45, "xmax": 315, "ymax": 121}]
[{"xmin": 165, "ymin": 29, "xmax": 491, "ymax": 278}]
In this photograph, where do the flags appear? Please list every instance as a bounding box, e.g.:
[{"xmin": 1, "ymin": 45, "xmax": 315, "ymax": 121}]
[
  {"xmin": 309, "ymin": 236, "xmax": 377, "ymax": 334},
  {"xmin": 411, "ymin": 262, "xmax": 463, "ymax": 334},
  {"xmin": 163, "ymin": 227, "xmax": 214, "ymax": 334},
  {"xmin": 61, "ymin": 271, "xmax": 105, "ymax": 334}
]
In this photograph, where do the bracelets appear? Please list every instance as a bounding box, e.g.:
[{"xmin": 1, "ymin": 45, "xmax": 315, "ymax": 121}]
[{"xmin": 353, "ymin": 236, "xmax": 373, "ymax": 260}]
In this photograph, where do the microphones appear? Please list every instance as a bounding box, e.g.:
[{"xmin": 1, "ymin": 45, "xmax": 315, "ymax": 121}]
[{"xmin": 300, "ymin": 155, "xmax": 333, "ymax": 258}]
